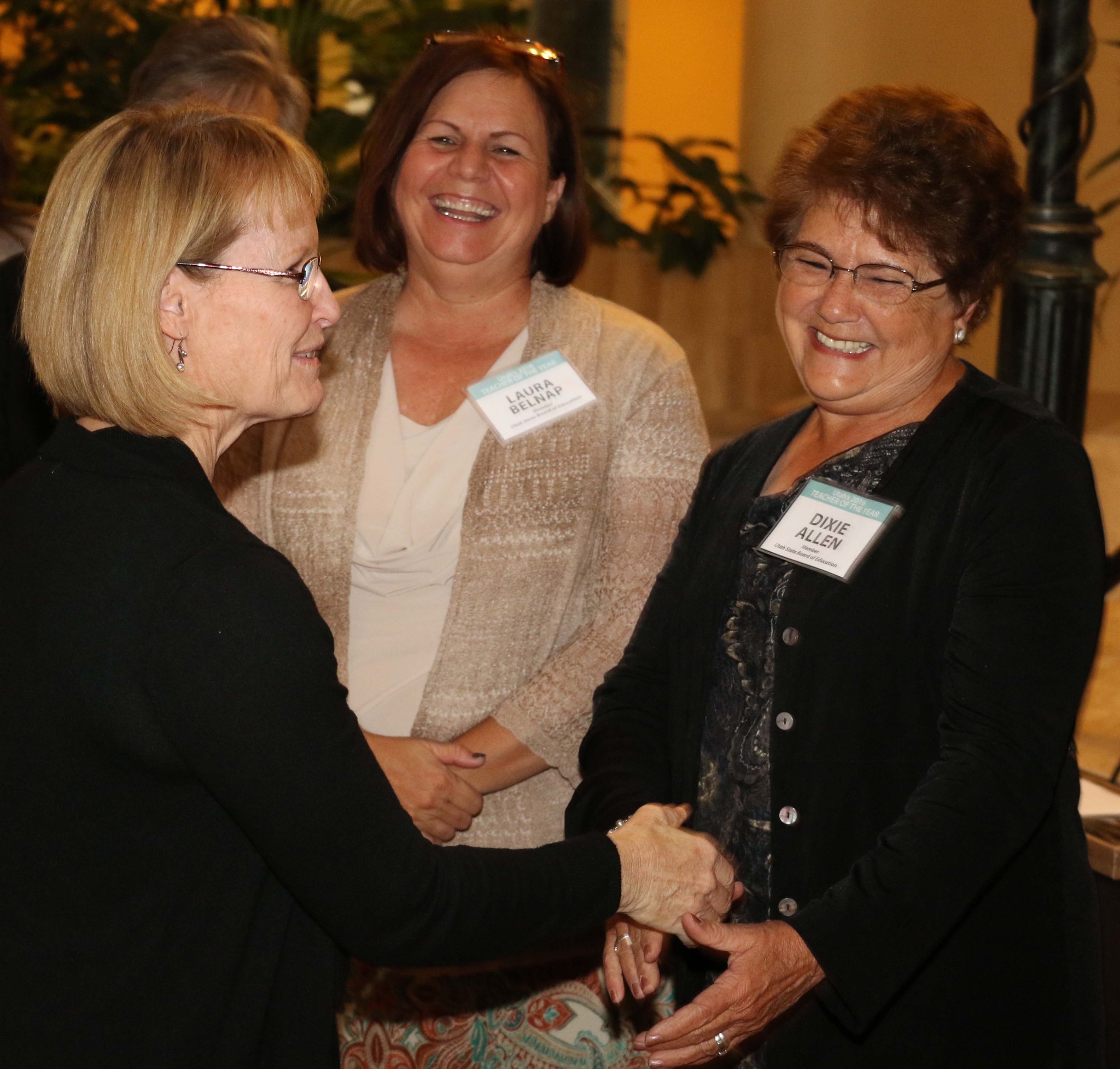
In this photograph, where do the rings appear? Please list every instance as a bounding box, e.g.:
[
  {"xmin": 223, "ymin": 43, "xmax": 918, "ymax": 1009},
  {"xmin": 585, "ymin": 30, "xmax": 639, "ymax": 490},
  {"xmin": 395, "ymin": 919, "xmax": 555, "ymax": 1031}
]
[
  {"xmin": 714, "ymin": 1033, "xmax": 729, "ymax": 1056},
  {"xmin": 614, "ymin": 934, "xmax": 628, "ymax": 948}
]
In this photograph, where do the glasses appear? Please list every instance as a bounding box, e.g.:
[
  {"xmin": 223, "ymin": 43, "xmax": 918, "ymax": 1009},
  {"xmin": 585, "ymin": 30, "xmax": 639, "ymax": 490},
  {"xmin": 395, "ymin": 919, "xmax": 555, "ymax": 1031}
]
[
  {"xmin": 425, "ymin": 30, "xmax": 565, "ymax": 83},
  {"xmin": 773, "ymin": 246, "xmax": 951, "ymax": 304},
  {"xmin": 173, "ymin": 252, "xmax": 323, "ymax": 303}
]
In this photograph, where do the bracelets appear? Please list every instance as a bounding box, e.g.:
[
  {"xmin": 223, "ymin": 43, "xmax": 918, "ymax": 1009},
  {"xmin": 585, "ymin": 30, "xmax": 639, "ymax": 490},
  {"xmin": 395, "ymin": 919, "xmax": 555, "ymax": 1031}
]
[{"xmin": 607, "ymin": 815, "xmax": 632, "ymax": 836}]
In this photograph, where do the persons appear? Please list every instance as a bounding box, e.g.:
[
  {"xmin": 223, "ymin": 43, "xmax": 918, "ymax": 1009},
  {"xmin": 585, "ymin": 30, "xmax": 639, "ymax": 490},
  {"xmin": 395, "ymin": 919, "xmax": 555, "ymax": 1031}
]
[
  {"xmin": 564, "ymin": 84, "xmax": 1104, "ymax": 1069},
  {"xmin": 0, "ymin": 106, "xmax": 735, "ymax": 1069},
  {"xmin": 0, "ymin": 16, "xmax": 310, "ymax": 320},
  {"xmin": 217, "ymin": 33, "xmax": 711, "ymax": 1069}
]
